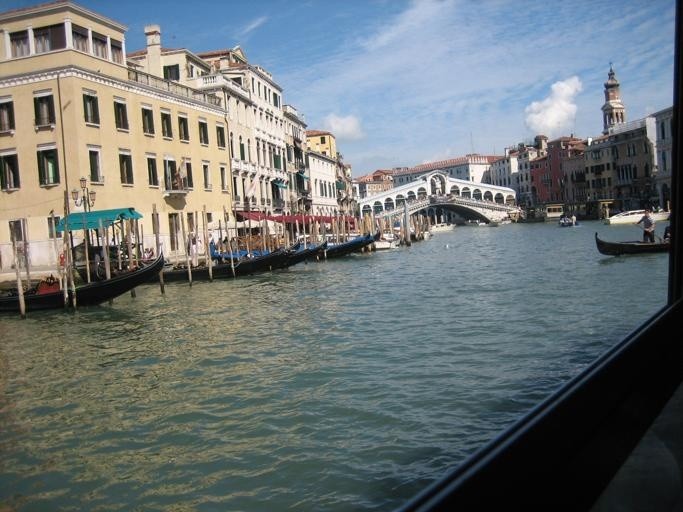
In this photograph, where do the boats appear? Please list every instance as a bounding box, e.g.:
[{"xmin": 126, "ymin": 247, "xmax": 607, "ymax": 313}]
[
  {"xmin": 596, "ymin": 232, "xmax": 670, "ymax": 255},
  {"xmin": 605, "ymin": 208, "xmax": 672, "ymax": 225},
  {"xmin": 559, "ymin": 218, "xmax": 578, "ymax": 227},
  {"xmin": 0, "ymin": 252, "xmax": 164, "ymax": 312}
]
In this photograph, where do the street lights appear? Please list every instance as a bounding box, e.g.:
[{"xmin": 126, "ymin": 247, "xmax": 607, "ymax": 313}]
[{"xmin": 72, "ymin": 178, "xmax": 96, "ymax": 244}]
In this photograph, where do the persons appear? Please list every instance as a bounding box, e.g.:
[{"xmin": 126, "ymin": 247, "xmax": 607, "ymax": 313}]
[{"xmin": 633, "ymin": 208, "xmax": 655, "ymax": 243}]
